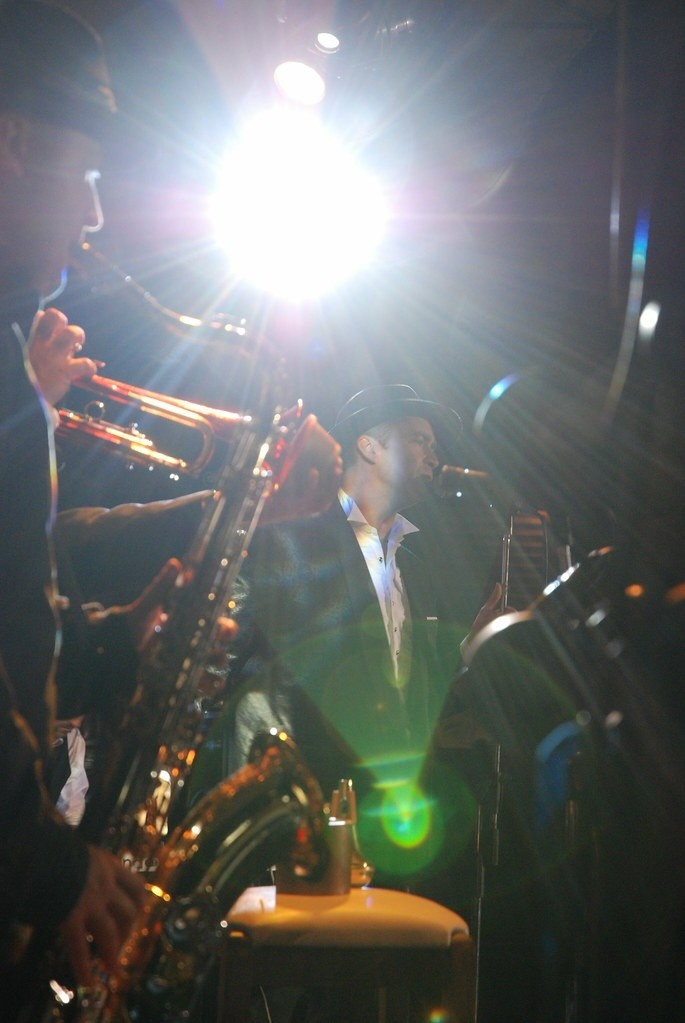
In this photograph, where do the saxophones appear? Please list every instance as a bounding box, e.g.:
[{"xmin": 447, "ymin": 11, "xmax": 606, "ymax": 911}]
[{"xmin": 36, "ymin": 214, "xmax": 304, "ymax": 1022}]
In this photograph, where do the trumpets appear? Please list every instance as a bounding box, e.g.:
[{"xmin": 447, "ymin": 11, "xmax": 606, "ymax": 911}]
[{"xmin": 55, "ymin": 370, "xmax": 309, "ymax": 478}]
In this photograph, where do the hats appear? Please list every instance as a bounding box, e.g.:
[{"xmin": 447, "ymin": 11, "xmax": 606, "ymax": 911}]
[
  {"xmin": 326, "ymin": 383, "xmax": 462, "ymax": 455},
  {"xmin": 1, "ymin": 1, "xmax": 168, "ymax": 159}
]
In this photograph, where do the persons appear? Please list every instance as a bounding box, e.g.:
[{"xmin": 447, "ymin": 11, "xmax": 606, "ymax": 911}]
[
  {"xmin": 235, "ymin": 380, "xmax": 518, "ymax": 889},
  {"xmin": 0, "ymin": 2, "xmax": 239, "ymax": 1021}
]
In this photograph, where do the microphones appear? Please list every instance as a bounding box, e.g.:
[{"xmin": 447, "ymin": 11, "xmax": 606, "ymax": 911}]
[{"xmin": 435, "ymin": 466, "xmax": 493, "ymax": 506}]
